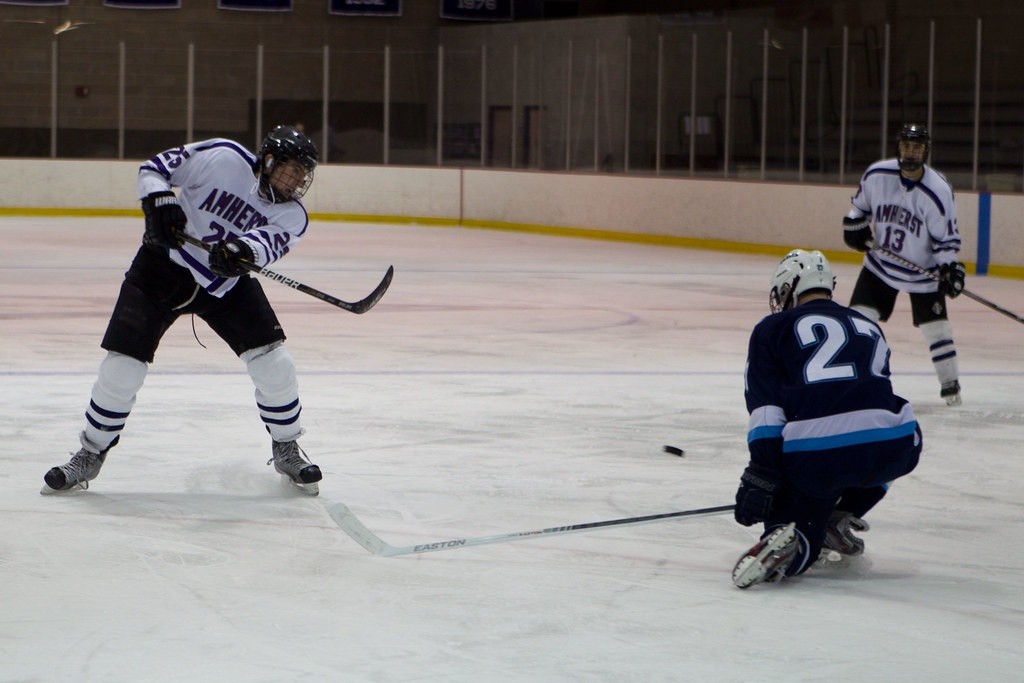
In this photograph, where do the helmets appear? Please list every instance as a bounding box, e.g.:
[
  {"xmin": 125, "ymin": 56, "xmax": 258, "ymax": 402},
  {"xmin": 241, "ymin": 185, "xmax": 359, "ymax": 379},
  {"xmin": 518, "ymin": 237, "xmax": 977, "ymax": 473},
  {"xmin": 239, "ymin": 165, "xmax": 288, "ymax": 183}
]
[
  {"xmin": 258, "ymin": 124, "xmax": 320, "ymax": 203},
  {"xmin": 895, "ymin": 124, "xmax": 931, "ymax": 172},
  {"xmin": 769, "ymin": 249, "xmax": 836, "ymax": 315}
]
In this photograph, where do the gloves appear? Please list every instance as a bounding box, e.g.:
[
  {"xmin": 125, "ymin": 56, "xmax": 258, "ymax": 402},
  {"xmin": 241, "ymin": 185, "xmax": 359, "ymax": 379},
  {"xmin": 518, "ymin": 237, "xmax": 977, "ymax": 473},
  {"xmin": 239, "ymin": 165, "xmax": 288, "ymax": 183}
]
[
  {"xmin": 208, "ymin": 240, "xmax": 254, "ymax": 278},
  {"xmin": 938, "ymin": 262, "xmax": 965, "ymax": 299},
  {"xmin": 142, "ymin": 191, "xmax": 187, "ymax": 249},
  {"xmin": 842, "ymin": 216, "xmax": 874, "ymax": 252},
  {"xmin": 735, "ymin": 461, "xmax": 784, "ymax": 527}
]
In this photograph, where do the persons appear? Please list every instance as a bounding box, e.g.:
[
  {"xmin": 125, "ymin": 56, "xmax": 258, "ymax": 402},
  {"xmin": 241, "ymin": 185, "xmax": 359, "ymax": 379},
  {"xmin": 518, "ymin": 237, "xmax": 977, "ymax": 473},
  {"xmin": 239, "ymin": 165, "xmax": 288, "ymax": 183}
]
[
  {"xmin": 40, "ymin": 124, "xmax": 323, "ymax": 496},
  {"xmin": 842, "ymin": 123, "xmax": 966, "ymax": 406},
  {"xmin": 730, "ymin": 248, "xmax": 923, "ymax": 591}
]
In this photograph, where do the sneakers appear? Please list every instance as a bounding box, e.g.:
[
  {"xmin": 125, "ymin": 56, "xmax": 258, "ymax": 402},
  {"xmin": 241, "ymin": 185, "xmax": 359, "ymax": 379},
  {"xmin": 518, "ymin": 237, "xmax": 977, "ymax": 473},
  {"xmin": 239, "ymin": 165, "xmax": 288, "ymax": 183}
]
[
  {"xmin": 40, "ymin": 434, "xmax": 120, "ymax": 494},
  {"xmin": 811, "ymin": 510, "xmax": 870, "ymax": 570},
  {"xmin": 266, "ymin": 425, "xmax": 322, "ymax": 496},
  {"xmin": 941, "ymin": 379, "xmax": 962, "ymax": 406},
  {"xmin": 732, "ymin": 522, "xmax": 799, "ymax": 589}
]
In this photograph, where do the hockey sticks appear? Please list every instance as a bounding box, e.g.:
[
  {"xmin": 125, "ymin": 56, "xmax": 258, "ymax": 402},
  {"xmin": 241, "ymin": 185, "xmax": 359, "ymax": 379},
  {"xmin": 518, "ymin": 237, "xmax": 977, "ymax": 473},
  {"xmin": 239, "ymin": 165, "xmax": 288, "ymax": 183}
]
[
  {"xmin": 325, "ymin": 501, "xmax": 736, "ymax": 559},
  {"xmin": 173, "ymin": 228, "xmax": 394, "ymax": 316},
  {"xmin": 866, "ymin": 240, "xmax": 1024, "ymax": 326}
]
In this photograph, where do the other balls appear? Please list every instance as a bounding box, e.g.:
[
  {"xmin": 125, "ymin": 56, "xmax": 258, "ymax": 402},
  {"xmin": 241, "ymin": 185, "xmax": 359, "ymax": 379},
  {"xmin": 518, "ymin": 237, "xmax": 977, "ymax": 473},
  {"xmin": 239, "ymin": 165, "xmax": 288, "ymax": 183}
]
[{"xmin": 663, "ymin": 444, "xmax": 683, "ymax": 458}]
